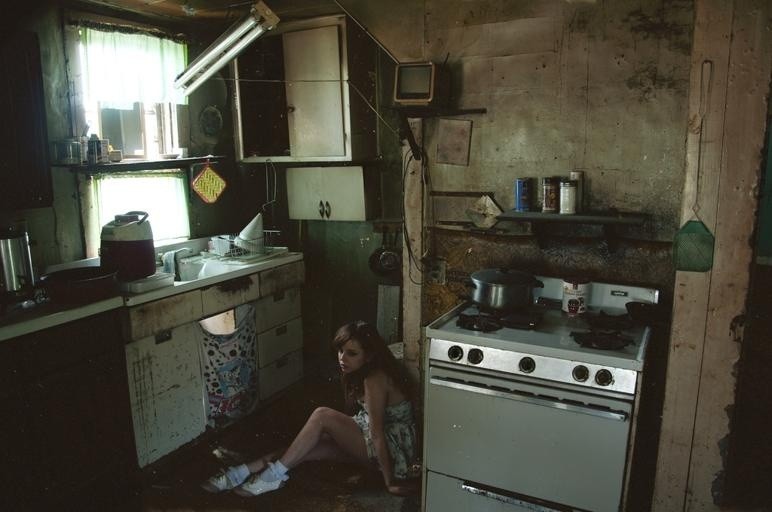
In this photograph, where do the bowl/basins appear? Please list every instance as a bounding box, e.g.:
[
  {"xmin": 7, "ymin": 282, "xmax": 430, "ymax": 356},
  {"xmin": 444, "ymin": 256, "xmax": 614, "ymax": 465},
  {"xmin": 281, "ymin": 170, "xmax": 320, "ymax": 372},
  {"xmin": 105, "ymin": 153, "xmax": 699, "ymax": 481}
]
[{"xmin": 39, "ymin": 265, "xmax": 120, "ymax": 303}]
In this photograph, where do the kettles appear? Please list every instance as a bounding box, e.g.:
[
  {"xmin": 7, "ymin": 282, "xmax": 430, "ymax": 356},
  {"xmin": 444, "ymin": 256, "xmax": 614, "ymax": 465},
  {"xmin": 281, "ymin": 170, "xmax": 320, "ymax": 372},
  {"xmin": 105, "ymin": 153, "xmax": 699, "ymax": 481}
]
[{"xmin": 1, "ymin": 226, "xmax": 38, "ymax": 302}]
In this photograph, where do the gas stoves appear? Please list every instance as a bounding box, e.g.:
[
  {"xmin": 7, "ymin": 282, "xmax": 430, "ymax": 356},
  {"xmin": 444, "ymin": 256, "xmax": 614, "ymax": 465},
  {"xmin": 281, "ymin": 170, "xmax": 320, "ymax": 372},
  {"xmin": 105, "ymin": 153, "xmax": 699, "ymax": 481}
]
[{"xmin": 428, "ymin": 300, "xmax": 651, "ymax": 403}]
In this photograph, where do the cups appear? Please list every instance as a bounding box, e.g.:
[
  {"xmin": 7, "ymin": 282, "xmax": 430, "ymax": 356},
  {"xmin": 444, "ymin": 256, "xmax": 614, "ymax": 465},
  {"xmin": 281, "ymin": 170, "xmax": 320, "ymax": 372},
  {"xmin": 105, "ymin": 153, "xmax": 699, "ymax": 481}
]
[
  {"xmin": 110, "ymin": 151, "xmax": 122, "ymax": 161},
  {"xmin": 48, "ymin": 141, "xmax": 82, "ymax": 167}
]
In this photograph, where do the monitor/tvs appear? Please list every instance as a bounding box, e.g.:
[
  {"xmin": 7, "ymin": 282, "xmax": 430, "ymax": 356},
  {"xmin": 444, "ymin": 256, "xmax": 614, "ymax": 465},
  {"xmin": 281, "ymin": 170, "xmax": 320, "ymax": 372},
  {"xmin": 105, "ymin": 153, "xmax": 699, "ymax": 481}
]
[{"xmin": 393, "ymin": 60, "xmax": 448, "ymax": 106}]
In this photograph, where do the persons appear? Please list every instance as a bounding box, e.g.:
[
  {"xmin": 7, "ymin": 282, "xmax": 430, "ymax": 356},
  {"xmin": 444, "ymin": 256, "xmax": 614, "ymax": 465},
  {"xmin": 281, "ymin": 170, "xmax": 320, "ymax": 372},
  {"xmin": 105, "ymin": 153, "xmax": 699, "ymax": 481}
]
[{"xmin": 199, "ymin": 318, "xmax": 421, "ymax": 504}]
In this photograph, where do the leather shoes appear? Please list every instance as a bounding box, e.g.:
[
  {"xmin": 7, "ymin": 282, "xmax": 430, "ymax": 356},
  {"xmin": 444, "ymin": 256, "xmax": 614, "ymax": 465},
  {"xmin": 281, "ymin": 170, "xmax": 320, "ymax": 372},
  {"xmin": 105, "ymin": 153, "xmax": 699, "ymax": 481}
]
[{"xmin": 198, "ymin": 465, "xmax": 282, "ymax": 499}]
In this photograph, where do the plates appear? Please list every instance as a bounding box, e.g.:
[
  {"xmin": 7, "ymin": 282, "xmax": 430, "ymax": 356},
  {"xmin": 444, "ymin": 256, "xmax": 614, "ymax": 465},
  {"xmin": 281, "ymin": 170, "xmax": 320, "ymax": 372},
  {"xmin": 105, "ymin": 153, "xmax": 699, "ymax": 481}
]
[{"xmin": 161, "ymin": 154, "xmax": 181, "ymax": 159}]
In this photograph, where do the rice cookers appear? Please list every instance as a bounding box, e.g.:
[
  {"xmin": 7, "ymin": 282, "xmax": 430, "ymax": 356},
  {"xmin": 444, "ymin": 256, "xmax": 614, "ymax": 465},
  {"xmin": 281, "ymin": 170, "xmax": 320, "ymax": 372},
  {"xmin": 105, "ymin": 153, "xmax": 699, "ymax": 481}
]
[{"xmin": 100, "ymin": 208, "xmax": 156, "ymax": 283}]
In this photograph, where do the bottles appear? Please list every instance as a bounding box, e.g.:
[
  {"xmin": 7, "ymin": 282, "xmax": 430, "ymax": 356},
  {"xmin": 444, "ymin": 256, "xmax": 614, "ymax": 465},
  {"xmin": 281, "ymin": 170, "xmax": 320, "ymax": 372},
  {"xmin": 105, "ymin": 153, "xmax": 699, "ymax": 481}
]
[
  {"xmin": 87, "ymin": 132, "xmax": 102, "ymax": 167},
  {"xmin": 540, "ymin": 172, "xmax": 583, "ymax": 216}
]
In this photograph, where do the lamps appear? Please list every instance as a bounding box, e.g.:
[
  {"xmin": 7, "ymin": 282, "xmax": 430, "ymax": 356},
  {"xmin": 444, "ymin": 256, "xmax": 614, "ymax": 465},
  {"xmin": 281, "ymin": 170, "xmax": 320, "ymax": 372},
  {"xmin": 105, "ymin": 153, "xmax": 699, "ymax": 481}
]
[{"xmin": 171, "ymin": 0, "xmax": 280, "ymax": 97}]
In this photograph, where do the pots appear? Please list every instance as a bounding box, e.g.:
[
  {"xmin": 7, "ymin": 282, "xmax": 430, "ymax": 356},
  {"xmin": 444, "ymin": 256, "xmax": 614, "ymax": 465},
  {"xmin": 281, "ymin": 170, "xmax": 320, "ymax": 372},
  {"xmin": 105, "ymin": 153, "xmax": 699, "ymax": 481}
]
[
  {"xmin": 464, "ymin": 266, "xmax": 544, "ymax": 315},
  {"xmin": 367, "ymin": 226, "xmax": 397, "ymax": 276}
]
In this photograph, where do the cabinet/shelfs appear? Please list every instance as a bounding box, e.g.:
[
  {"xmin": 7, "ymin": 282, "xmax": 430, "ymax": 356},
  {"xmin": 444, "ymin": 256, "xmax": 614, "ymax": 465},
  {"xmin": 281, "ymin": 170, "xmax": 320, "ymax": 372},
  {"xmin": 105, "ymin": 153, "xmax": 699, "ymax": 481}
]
[
  {"xmin": 122, "ymin": 260, "xmax": 307, "ymax": 470},
  {"xmin": 285, "ymin": 165, "xmax": 379, "ymax": 223},
  {"xmin": 229, "ymin": 13, "xmax": 384, "ymax": 167}
]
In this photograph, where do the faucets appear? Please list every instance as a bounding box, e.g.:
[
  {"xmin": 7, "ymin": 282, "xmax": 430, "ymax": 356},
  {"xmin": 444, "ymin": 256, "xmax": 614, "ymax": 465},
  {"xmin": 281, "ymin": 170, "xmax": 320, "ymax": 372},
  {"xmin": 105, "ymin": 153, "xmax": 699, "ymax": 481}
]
[{"xmin": 161, "ymin": 247, "xmax": 193, "ymax": 264}]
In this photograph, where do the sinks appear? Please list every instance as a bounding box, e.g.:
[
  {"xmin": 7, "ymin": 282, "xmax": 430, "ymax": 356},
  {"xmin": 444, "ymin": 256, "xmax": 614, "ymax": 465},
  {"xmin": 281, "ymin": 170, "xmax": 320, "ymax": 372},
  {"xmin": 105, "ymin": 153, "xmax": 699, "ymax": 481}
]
[{"xmin": 160, "ymin": 261, "xmax": 248, "ymax": 282}]
